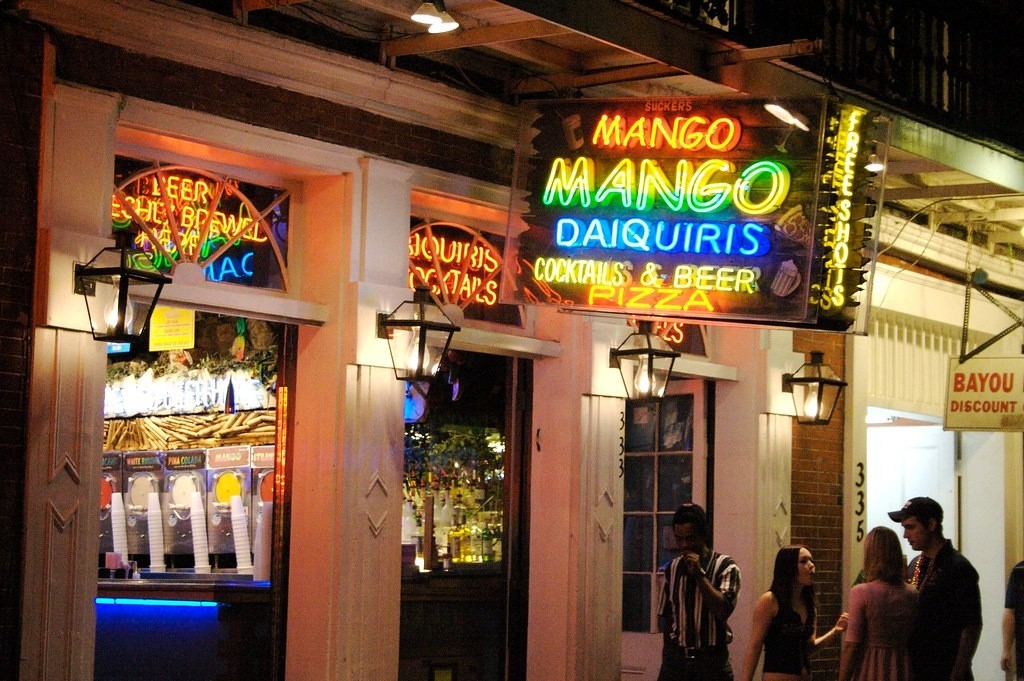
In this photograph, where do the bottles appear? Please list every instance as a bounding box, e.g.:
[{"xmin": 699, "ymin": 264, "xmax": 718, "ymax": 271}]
[
  {"xmin": 412, "ymin": 519, "xmax": 424, "ymax": 558},
  {"xmin": 447, "ymin": 514, "xmax": 494, "ymax": 562}
]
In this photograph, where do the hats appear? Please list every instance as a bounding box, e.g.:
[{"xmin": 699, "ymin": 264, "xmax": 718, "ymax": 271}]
[{"xmin": 888, "ymin": 496, "xmax": 944, "ymax": 523}]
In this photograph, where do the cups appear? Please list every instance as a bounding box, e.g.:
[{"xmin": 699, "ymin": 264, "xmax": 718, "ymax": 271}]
[
  {"xmin": 190, "ymin": 492, "xmax": 211, "ymax": 573},
  {"xmin": 231, "ymin": 496, "xmax": 273, "ymax": 582},
  {"xmin": 105, "ymin": 552, "xmax": 122, "ymax": 567},
  {"xmin": 111, "ymin": 492, "xmax": 129, "ymax": 567},
  {"xmin": 147, "ymin": 492, "xmax": 166, "ymax": 572}
]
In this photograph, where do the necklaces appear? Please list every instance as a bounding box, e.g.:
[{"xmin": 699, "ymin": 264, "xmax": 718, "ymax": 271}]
[{"xmin": 911, "ymin": 538, "xmax": 947, "ymax": 593}]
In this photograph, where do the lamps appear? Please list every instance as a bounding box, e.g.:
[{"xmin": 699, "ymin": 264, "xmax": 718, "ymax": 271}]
[
  {"xmin": 375, "ymin": 285, "xmax": 462, "ymax": 385},
  {"xmin": 72, "ymin": 228, "xmax": 175, "ymax": 345},
  {"xmin": 607, "ymin": 319, "xmax": 683, "ymax": 407},
  {"xmin": 780, "ymin": 349, "xmax": 850, "ymax": 428},
  {"xmin": 410, "ymin": 0, "xmax": 461, "ymax": 33},
  {"xmin": 864, "ymin": 153, "xmax": 884, "ymax": 172}
]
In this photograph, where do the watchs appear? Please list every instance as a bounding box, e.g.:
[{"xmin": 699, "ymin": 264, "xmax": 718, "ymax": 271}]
[{"xmin": 697, "ymin": 568, "xmax": 707, "ymax": 577}]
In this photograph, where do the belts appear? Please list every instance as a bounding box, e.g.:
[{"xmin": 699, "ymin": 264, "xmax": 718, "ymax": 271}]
[{"xmin": 675, "ymin": 646, "xmax": 721, "ymax": 659}]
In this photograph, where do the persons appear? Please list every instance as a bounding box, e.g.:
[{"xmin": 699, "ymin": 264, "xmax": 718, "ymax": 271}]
[
  {"xmin": 651, "ymin": 503, "xmax": 741, "ymax": 681},
  {"xmin": 741, "ymin": 497, "xmax": 1024, "ymax": 681}
]
